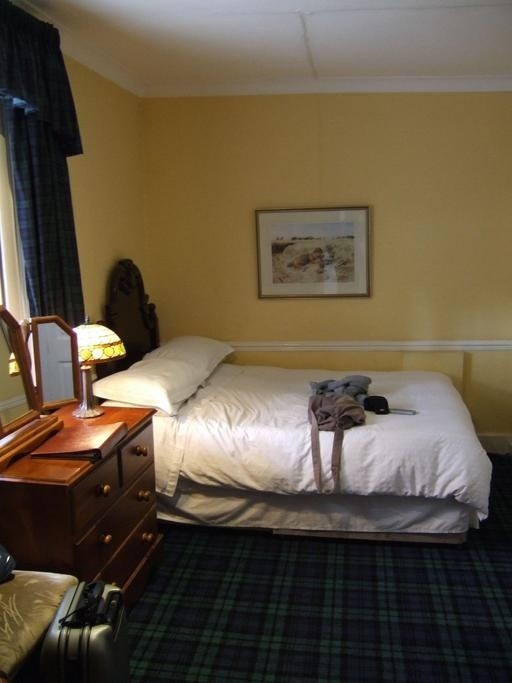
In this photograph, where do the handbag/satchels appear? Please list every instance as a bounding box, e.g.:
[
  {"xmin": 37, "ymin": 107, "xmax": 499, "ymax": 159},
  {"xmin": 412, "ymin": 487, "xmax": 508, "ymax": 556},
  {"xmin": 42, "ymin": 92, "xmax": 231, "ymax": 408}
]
[{"xmin": 308, "ymin": 394, "xmax": 365, "ymax": 496}]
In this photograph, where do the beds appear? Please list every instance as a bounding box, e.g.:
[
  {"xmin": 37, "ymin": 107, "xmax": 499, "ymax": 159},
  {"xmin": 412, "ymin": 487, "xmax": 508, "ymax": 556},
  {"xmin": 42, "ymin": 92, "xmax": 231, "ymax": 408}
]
[{"xmin": 98, "ymin": 363, "xmax": 468, "ymax": 546}]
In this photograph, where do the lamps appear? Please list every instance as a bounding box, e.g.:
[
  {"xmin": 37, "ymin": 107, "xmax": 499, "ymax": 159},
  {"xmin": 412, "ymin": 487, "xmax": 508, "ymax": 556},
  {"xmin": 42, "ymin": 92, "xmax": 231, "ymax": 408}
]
[{"xmin": 73, "ymin": 315, "xmax": 126, "ymax": 418}]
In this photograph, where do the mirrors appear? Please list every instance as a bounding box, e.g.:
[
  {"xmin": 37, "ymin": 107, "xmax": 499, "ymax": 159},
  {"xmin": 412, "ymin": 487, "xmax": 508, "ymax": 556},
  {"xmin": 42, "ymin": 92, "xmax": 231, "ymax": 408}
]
[{"xmin": 0, "ymin": 306, "xmax": 83, "ymax": 438}]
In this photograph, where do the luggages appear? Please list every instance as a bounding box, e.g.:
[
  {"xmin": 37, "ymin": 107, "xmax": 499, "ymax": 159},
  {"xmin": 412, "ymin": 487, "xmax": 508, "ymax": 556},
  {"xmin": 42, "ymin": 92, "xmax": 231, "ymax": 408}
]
[{"xmin": 39, "ymin": 580, "xmax": 129, "ymax": 682}]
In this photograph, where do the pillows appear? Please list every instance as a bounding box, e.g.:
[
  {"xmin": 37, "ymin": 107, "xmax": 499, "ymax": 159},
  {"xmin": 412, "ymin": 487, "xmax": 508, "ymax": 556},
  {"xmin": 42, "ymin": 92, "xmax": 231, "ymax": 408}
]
[{"xmin": 90, "ymin": 335, "xmax": 235, "ymax": 415}]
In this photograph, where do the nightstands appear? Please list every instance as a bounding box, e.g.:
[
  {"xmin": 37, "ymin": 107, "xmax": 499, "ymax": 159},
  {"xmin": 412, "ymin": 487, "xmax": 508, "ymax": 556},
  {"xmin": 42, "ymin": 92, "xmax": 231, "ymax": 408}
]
[{"xmin": 0, "ymin": 405, "xmax": 165, "ymax": 593}]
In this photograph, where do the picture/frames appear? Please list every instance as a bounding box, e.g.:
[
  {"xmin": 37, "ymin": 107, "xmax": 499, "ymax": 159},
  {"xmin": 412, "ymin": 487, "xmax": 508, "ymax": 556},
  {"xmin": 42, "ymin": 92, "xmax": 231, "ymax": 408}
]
[{"xmin": 254, "ymin": 206, "xmax": 371, "ymax": 298}]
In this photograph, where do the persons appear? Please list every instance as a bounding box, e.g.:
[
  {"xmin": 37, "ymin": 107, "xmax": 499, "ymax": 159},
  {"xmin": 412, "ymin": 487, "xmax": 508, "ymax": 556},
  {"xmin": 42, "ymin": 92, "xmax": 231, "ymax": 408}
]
[{"xmin": 287, "ymin": 246, "xmax": 323, "ymax": 269}]
